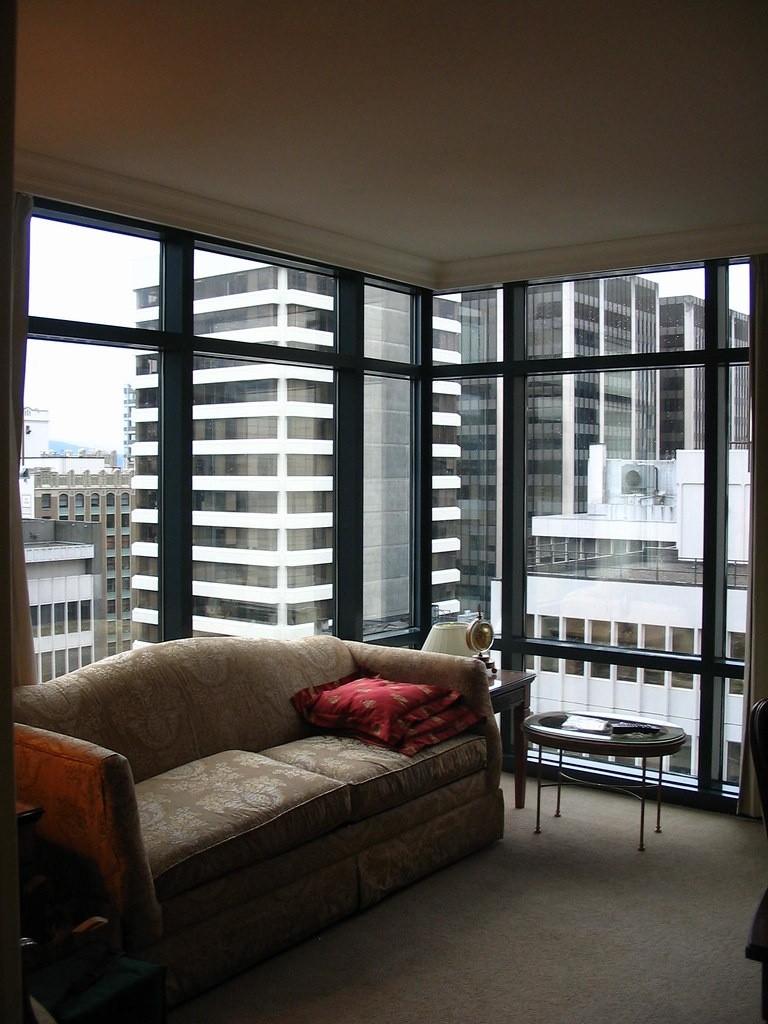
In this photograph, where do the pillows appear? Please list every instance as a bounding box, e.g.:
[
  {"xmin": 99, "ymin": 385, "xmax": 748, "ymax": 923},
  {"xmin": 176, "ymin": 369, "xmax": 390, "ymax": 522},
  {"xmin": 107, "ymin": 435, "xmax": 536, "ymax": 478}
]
[
  {"xmin": 289, "ymin": 671, "xmax": 462, "ymax": 748},
  {"xmin": 357, "ymin": 699, "xmax": 490, "ymax": 758}
]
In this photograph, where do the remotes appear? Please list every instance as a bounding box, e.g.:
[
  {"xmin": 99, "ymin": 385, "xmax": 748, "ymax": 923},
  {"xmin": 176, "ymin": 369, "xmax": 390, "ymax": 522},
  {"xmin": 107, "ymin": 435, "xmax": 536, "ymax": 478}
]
[{"xmin": 611, "ymin": 724, "xmax": 660, "ymax": 735}]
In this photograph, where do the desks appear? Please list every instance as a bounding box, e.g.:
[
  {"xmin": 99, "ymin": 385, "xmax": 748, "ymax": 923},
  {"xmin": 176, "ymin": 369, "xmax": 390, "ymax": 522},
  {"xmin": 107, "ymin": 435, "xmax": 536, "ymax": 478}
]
[{"xmin": 521, "ymin": 710, "xmax": 687, "ymax": 852}]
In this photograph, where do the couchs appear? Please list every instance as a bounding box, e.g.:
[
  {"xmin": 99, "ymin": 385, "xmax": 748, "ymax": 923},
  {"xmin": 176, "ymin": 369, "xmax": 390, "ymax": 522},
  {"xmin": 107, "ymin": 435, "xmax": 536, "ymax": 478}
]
[{"xmin": 11, "ymin": 635, "xmax": 506, "ymax": 1012}]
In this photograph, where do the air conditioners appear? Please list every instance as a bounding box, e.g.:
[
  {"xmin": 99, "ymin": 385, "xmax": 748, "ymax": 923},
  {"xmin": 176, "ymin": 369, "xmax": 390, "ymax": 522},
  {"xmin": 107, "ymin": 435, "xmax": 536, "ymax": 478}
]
[{"xmin": 621, "ymin": 461, "xmax": 658, "ymax": 495}]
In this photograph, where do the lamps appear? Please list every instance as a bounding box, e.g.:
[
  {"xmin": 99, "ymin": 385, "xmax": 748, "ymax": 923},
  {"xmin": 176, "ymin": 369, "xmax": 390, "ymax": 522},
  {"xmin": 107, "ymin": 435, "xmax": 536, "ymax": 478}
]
[{"xmin": 421, "ymin": 621, "xmax": 471, "ymax": 659}]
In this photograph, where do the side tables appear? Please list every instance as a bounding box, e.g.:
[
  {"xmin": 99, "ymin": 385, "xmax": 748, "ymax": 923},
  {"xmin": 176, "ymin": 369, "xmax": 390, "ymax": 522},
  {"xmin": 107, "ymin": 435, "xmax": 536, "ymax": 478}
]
[{"xmin": 489, "ymin": 669, "xmax": 536, "ymax": 809}]
